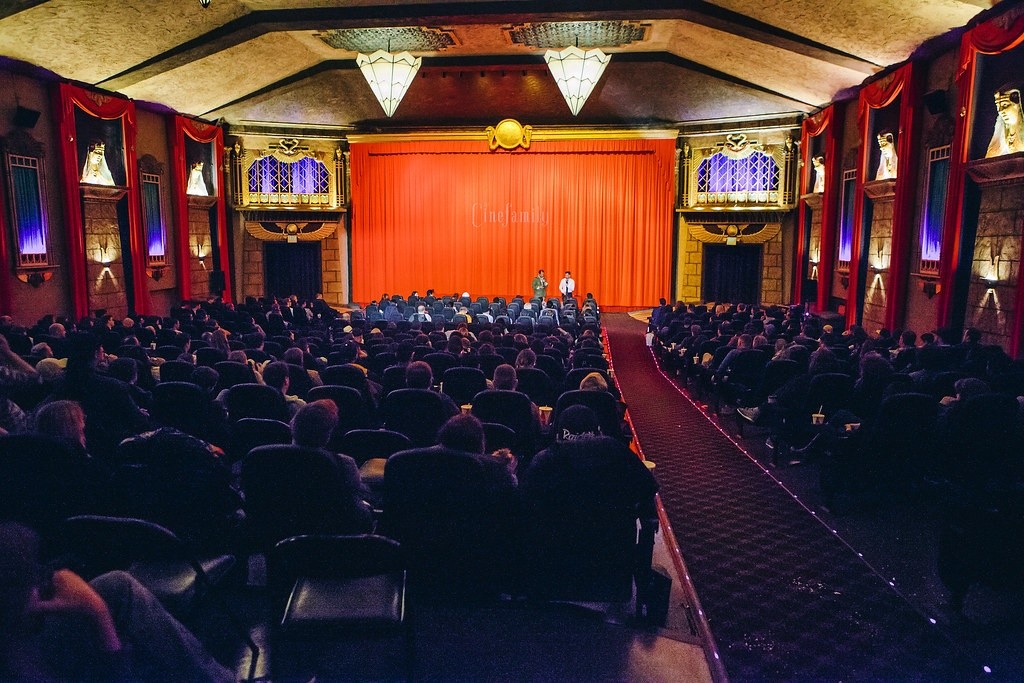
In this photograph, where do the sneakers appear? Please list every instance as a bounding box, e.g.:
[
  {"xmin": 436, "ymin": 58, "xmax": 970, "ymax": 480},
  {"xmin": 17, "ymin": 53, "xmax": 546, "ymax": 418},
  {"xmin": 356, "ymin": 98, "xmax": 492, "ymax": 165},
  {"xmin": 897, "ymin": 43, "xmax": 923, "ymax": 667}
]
[
  {"xmin": 737, "ymin": 407, "xmax": 760, "ymax": 423},
  {"xmin": 765, "ymin": 438, "xmax": 775, "ymax": 449}
]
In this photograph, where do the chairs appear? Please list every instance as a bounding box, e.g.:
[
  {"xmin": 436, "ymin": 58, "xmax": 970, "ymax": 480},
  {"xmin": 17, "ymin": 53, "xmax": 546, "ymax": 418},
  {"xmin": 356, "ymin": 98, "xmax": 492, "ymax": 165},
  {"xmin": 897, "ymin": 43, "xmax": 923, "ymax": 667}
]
[
  {"xmin": 0, "ymin": 296, "xmax": 654, "ymax": 652},
  {"xmin": 646, "ymin": 303, "xmax": 1024, "ymax": 502}
]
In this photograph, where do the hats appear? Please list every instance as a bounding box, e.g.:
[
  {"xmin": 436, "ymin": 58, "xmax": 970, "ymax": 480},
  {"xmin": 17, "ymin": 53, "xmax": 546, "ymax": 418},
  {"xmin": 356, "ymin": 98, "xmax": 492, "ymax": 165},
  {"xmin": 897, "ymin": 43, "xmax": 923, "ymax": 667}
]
[
  {"xmin": 343, "ymin": 325, "xmax": 352, "ymax": 333},
  {"xmin": 418, "ymin": 306, "xmax": 425, "ymax": 315},
  {"xmin": 370, "ymin": 328, "xmax": 381, "ymax": 333},
  {"xmin": 954, "ymin": 377, "xmax": 995, "ymax": 402}
]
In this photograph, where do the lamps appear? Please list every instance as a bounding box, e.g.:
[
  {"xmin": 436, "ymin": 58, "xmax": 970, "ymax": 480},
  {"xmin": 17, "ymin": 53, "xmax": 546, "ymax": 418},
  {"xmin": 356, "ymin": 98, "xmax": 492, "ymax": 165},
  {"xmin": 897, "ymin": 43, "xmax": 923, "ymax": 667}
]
[
  {"xmin": 980, "ymin": 265, "xmax": 999, "ymax": 288},
  {"xmin": 102, "ymin": 261, "xmax": 111, "ymax": 267},
  {"xmin": 357, "ymin": 39, "xmax": 422, "ymax": 118},
  {"xmin": 543, "ymin": 35, "xmax": 612, "ymax": 117},
  {"xmin": 869, "ymin": 265, "xmax": 883, "ymax": 280},
  {"xmin": 198, "ymin": 256, "xmax": 206, "ymax": 261}
]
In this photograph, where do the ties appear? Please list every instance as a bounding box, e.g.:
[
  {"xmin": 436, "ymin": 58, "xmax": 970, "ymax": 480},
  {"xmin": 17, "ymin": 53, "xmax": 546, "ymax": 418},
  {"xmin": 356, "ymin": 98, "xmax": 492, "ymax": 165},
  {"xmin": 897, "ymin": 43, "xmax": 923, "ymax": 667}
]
[{"xmin": 566, "ymin": 279, "xmax": 568, "ymax": 295}]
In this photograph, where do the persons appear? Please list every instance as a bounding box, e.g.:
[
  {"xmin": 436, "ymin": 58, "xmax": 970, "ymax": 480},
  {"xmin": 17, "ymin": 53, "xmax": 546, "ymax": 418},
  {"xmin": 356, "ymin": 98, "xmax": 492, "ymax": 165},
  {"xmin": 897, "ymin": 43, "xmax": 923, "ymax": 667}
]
[
  {"xmin": 532, "ymin": 270, "xmax": 548, "ymax": 298},
  {"xmin": 651, "ymin": 298, "xmax": 1024, "ymax": 461},
  {"xmin": 0, "ymin": 284, "xmax": 608, "ymax": 683},
  {"xmin": 559, "ymin": 272, "xmax": 575, "ymax": 301}
]
